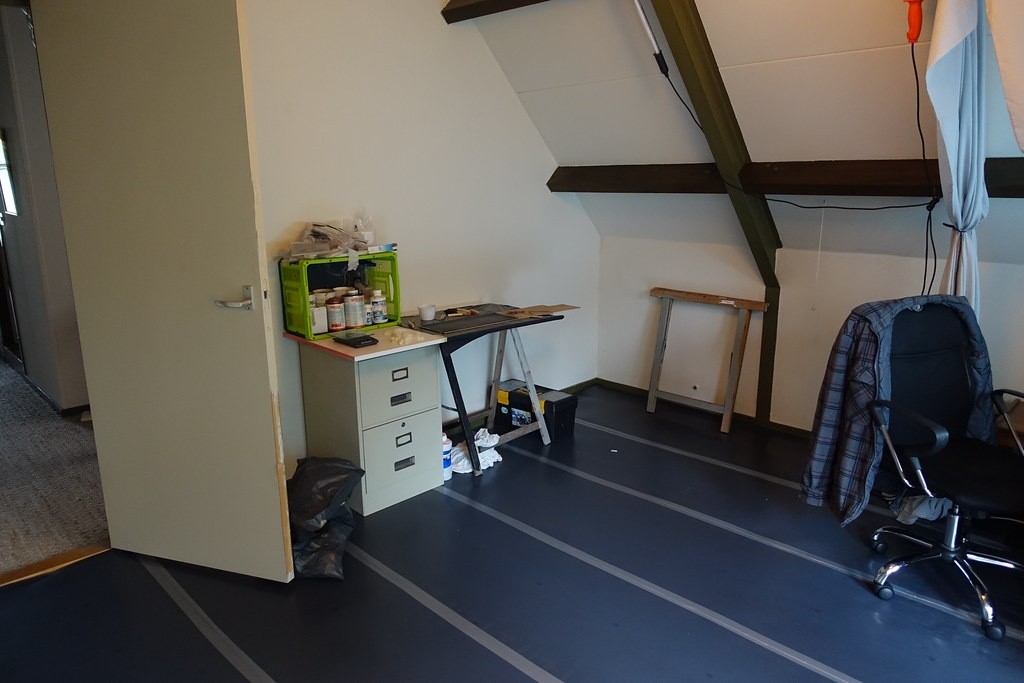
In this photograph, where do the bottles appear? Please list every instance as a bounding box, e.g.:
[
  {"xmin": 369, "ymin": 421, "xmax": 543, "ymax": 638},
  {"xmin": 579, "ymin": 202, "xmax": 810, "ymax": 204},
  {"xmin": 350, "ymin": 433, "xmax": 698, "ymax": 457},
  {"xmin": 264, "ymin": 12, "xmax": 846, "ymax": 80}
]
[
  {"xmin": 442, "ymin": 433, "xmax": 453, "ymax": 481},
  {"xmin": 310, "ymin": 284, "xmax": 387, "ymax": 336}
]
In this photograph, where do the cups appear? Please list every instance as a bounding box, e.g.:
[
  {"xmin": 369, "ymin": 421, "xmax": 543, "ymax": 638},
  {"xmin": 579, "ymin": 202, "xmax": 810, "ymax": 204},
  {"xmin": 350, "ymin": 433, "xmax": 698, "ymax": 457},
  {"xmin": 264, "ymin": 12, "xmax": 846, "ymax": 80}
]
[{"xmin": 417, "ymin": 304, "xmax": 436, "ymax": 320}]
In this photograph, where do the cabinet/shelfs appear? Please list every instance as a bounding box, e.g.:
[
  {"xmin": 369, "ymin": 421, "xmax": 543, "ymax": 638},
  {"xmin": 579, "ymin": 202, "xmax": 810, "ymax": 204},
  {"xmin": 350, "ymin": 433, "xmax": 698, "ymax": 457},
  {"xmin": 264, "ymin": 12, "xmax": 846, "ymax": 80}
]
[{"xmin": 283, "ymin": 328, "xmax": 446, "ymax": 518}]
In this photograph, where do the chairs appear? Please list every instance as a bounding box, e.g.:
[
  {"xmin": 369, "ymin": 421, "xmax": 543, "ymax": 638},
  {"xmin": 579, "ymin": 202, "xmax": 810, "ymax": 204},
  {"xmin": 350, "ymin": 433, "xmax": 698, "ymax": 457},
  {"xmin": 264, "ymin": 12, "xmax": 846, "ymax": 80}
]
[{"xmin": 848, "ymin": 293, "xmax": 1024, "ymax": 643}]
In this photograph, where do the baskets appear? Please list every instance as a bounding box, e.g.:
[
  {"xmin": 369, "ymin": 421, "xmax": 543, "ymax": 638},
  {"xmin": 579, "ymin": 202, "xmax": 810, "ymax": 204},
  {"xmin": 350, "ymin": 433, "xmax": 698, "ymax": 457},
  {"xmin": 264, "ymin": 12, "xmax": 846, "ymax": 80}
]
[{"xmin": 279, "ymin": 251, "xmax": 401, "ymax": 341}]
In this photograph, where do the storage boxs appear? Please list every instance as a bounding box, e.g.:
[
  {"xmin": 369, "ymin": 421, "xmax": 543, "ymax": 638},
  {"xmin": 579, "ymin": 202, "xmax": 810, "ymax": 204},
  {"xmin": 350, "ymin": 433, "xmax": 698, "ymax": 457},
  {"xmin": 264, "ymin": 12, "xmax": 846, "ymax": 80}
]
[
  {"xmin": 487, "ymin": 378, "xmax": 579, "ymax": 455},
  {"xmin": 279, "ymin": 250, "xmax": 402, "ymax": 340}
]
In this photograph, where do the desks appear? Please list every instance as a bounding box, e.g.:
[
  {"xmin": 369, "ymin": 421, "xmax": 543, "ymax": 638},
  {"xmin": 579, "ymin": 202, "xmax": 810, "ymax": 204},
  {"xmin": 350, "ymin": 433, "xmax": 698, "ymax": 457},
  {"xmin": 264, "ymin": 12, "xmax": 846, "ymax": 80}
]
[{"xmin": 395, "ymin": 303, "xmax": 564, "ymax": 477}]
